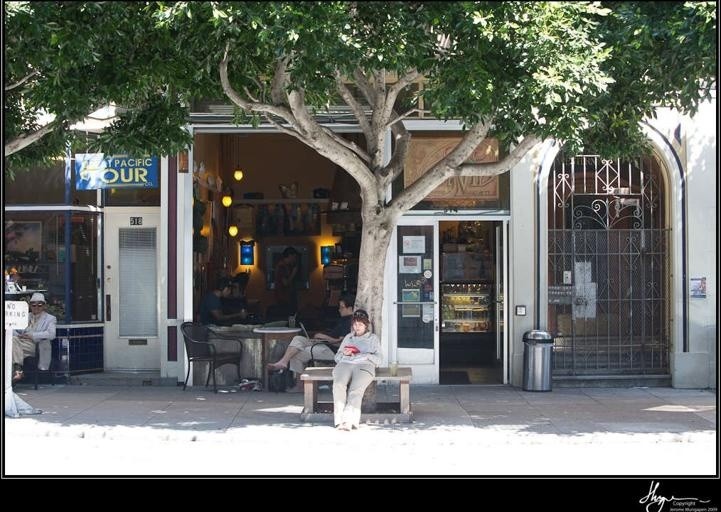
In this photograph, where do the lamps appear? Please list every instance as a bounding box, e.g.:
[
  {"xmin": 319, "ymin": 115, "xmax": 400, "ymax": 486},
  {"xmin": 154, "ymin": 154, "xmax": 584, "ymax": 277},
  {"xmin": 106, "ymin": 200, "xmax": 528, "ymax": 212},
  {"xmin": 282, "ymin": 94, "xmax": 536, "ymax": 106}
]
[
  {"xmin": 240, "ymin": 240, "xmax": 255, "ymax": 264},
  {"xmin": 228, "ymin": 195, "xmax": 239, "ymax": 237},
  {"xmin": 222, "ymin": 147, "xmax": 232, "ymax": 207},
  {"xmin": 320, "ymin": 245, "xmax": 334, "ymax": 266},
  {"xmin": 233, "ymin": 133, "xmax": 244, "ymax": 181}
]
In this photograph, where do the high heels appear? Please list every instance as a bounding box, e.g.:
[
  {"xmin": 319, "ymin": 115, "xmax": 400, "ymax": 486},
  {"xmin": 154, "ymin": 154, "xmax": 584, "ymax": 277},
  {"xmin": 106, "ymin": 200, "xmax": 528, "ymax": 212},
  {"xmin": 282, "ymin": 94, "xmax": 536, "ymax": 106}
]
[{"xmin": 267, "ymin": 362, "xmax": 288, "ymax": 375}]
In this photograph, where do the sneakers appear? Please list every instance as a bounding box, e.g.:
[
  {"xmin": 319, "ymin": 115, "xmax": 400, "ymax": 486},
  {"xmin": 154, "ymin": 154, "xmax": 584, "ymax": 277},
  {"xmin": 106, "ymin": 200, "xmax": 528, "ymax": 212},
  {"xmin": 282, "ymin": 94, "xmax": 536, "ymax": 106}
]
[
  {"xmin": 13, "ymin": 370, "xmax": 24, "ymax": 381},
  {"xmin": 288, "ymin": 385, "xmax": 304, "ymax": 392}
]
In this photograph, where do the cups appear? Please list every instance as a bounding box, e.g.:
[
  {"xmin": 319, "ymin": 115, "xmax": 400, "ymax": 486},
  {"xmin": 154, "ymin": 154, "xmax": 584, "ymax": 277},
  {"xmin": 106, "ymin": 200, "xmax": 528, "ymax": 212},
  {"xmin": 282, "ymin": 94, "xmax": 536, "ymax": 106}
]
[
  {"xmin": 390, "ymin": 361, "xmax": 400, "ymax": 376},
  {"xmin": 289, "ymin": 316, "xmax": 296, "ymax": 329}
]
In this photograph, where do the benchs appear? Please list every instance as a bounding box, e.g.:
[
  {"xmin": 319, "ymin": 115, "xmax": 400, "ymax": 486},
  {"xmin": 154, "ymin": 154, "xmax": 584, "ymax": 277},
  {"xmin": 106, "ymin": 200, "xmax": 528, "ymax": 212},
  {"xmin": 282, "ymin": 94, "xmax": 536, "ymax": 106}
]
[{"xmin": 301, "ymin": 365, "xmax": 412, "ymax": 417}]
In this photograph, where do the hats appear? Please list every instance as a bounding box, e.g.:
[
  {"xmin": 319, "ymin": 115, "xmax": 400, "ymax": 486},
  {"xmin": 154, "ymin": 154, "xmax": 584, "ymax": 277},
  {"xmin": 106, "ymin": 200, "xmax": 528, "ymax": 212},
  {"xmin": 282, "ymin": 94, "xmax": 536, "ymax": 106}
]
[{"xmin": 30, "ymin": 293, "xmax": 46, "ymax": 304}]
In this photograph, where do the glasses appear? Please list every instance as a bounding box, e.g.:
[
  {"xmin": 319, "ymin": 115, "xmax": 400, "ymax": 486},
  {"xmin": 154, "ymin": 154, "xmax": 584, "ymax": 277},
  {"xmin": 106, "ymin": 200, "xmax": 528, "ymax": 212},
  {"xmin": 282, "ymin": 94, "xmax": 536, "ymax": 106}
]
[
  {"xmin": 354, "ymin": 312, "xmax": 365, "ymax": 318},
  {"xmin": 31, "ymin": 303, "xmax": 42, "ymax": 307}
]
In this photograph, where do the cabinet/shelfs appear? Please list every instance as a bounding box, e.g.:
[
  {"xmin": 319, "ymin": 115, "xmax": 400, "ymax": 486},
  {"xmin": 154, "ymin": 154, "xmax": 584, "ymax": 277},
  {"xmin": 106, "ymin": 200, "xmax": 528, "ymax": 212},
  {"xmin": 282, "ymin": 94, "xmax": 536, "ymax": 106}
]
[
  {"xmin": 440, "ymin": 284, "xmax": 491, "ymax": 332},
  {"xmin": 6, "ymin": 240, "xmax": 98, "ymax": 320}
]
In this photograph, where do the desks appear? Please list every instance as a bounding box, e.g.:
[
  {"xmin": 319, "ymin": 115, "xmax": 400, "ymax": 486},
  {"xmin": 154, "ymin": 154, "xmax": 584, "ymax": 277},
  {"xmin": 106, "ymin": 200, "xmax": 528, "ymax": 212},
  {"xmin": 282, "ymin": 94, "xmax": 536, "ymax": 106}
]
[{"xmin": 216, "ymin": 328, "xmax": 301, "ymax": 393}]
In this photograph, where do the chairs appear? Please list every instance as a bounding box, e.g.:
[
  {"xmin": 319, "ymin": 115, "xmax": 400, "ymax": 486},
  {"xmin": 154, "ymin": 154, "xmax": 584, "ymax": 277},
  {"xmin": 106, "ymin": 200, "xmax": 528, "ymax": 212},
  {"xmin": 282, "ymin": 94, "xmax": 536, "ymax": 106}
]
[
  {"xmin": 303, "ymin": 343, "xmax": 341, "ymax": 395},
  {"xmin": 11, "ymin": 336, "xmax": 60, "ymax": 393},
  {"xmin": 181, "ymin": 322, "xmax": 244, "ymax": 393}
]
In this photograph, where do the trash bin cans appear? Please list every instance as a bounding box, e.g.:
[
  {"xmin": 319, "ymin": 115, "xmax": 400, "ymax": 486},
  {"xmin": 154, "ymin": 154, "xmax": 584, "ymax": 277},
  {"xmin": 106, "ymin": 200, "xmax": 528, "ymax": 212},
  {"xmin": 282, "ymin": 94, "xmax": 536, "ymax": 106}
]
[{"xmin": 522, "ymin": 329, "xmax": 554, "ymax": 392}]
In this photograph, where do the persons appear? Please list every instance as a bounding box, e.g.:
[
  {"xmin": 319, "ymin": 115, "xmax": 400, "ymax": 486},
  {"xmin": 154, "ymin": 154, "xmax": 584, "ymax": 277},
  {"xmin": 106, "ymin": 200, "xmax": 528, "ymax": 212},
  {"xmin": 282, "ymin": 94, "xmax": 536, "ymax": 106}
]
[
  {"xmin": 11, "ymin": 293, "xmax": 58, "ymax": 381},
  {"xmin": 701, "ymin": 278, "xmax": 707, "ymax": 295},
  {"xmin": 275, "ymin": 248, "xmax": 301, "ymax": 320},
  {"xmin": 199, "ymin": 271, "xmax": 261, "ymax": 325},
  {"xmin": 269, "ymin": 293, "xmax": 357, "ymax": 394},
  {"xmin": 331, "ymin": 308, "xmax": 383, "ymax": 431}
]
